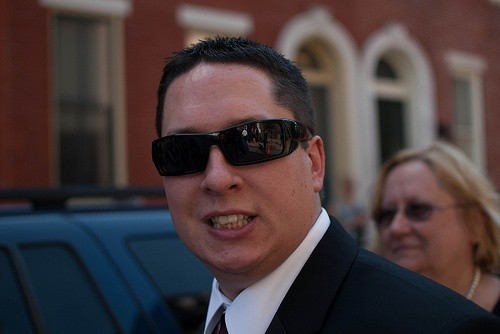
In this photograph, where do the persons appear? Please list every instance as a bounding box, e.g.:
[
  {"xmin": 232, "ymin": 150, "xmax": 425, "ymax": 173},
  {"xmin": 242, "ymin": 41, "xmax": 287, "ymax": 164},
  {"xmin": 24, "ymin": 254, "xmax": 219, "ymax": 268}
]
[
  {"xmin": 371, "ymin": 144, "xmax": 499, "ymax": 316},
  {"xmin": 145, "ymin": 32, "xmax": 500, "ymax": 333}
]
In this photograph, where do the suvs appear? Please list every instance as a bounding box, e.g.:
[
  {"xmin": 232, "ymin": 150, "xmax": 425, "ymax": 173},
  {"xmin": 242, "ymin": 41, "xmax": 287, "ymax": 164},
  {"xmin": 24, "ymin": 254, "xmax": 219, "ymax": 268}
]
[{"xmin": 1, "ymin": 185, "xmax": 215, "ymax": 334}]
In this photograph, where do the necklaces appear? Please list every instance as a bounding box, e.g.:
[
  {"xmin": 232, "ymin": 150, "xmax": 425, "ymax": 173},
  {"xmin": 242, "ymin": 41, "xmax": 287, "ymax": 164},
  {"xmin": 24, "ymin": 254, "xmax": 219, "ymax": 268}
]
[{"xmin": 461, "ymin": 265, "xmax": 483, "ymax": 301}]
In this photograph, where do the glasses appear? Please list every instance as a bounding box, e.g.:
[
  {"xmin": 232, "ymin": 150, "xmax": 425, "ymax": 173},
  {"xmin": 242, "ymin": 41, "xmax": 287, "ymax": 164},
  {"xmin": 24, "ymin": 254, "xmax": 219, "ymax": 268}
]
[
  {"xmin": 372, "ymin": 201, "xmax": 470, "ymax": 227},
  {"xmin": 151, "ymin": 117, "xmax": 315, "ymax": 177}
]
